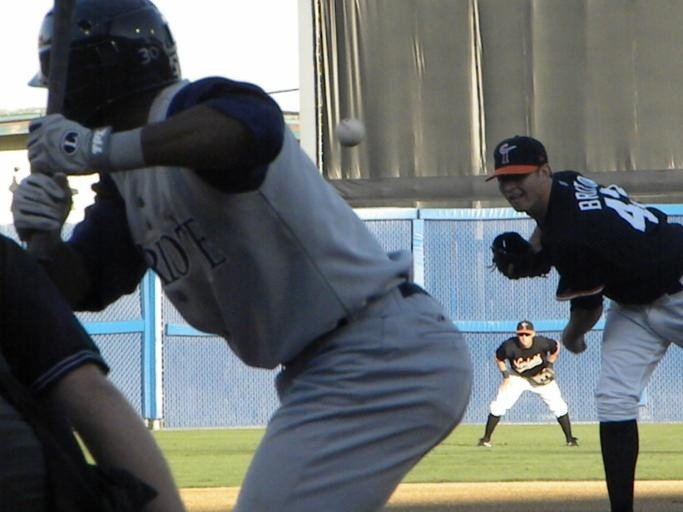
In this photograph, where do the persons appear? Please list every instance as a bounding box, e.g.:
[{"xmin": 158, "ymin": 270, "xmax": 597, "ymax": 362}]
[
  {"xmin": 1, "ymin": 235, "xmax": 185, "ymax": 511},
  {"xmin": 478, "ymin": 320, "xmax": 579, "ymax": 446},
  {"xmin": 11, "ymin": 0, "xmax": 470, "ymax": 510},
  {"xmin": 485, "ymin": 135, "xmax": 683, "ymax": 512}
]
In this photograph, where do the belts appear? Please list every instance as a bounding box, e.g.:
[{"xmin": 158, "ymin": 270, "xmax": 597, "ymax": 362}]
[{"xmin": 284, "ymin": 276, "xmax": 428, "ymax": 367}]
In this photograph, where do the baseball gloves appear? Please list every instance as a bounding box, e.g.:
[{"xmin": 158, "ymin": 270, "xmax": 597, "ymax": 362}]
[
  {"xmin": 492, "ymin": 231, "xmax": 539, "ymax": 280},
  {"xmin": 533, "ymin": 366, "xmax": 555, "ymax": 385}
]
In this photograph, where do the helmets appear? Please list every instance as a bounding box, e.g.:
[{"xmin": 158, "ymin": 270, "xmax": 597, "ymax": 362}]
[{"xmin": 27, "ymin": 1, "xmax": 183, "ymax": 116}]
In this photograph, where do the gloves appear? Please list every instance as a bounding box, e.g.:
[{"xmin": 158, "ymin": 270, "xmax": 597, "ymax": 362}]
[
  {"xmin": 11, "ymin": 174, "xmax": 73, "ymax": 240},
  {"xmin": 26, "ymin": 112, "xmax": 112, "ymax": 176}
]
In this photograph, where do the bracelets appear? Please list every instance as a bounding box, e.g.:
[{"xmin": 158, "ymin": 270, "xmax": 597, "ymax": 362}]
[
  {"xmin": 546, "ymin": 361, "xmax": 553, "ymax": 369},
  {"xmin": 501, "ymin": 370, "xmax": 509, "ymax": 378}
]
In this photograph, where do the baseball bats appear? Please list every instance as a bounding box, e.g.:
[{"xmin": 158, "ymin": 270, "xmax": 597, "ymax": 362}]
[{"xmin": 27, "ymin": 1, "xmax": 77, "ymax": 263}]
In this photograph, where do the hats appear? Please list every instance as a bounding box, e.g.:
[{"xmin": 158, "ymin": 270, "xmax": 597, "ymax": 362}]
[
  {"xmin": 515, "ymin": 321, "xmax": 535, "ymax": 335},
  {"xmin": 482, "ymin": 134, "xmax": 547, "ymax": 183}
]
